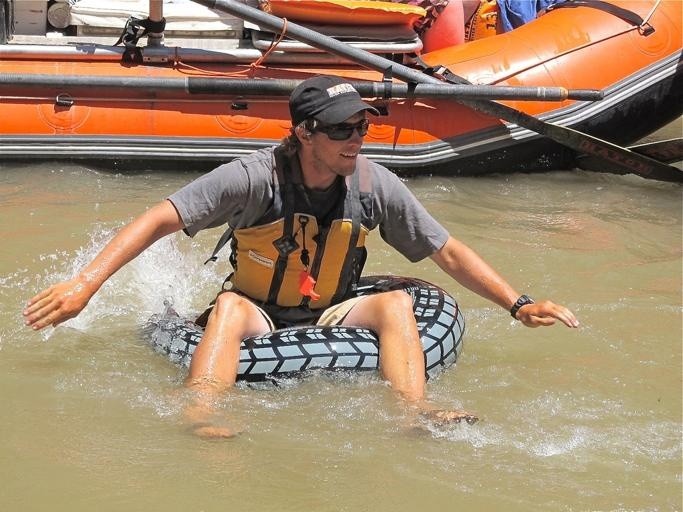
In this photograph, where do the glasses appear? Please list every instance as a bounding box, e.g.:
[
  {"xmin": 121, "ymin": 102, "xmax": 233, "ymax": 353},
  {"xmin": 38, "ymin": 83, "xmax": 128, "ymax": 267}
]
[{"xmin": 301, "ymin": 117, "xmax": 369, "ymax": 140}]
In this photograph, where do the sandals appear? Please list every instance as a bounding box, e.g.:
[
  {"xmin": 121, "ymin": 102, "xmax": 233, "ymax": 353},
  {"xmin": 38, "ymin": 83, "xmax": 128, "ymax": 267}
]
[
  {"xmin": 400, "ymin": 408, "xmax": 480, "ymax": 442},
  {"xmin": 168, "ymin": 416, "xmax": 243, "ymax": 440}
]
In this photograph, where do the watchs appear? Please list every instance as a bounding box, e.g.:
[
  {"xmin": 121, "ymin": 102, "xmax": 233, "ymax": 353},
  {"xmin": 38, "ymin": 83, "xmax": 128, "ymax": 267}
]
[{"xmin": 509, "ymin": 294, "xmax": 534, "ymax": 321}]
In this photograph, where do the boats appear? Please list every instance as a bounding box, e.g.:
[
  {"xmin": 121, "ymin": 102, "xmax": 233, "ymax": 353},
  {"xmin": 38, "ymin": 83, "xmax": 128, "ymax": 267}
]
[{"xmin": 0, "ymin": 0, "xmax": 682, "ymax": 179}]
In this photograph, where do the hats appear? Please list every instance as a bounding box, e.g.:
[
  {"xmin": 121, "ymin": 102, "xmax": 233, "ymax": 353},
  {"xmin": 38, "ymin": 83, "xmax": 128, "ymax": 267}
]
[{"xmin": 287, "ymin": 74, "xmax": 380, "ymax": 125}]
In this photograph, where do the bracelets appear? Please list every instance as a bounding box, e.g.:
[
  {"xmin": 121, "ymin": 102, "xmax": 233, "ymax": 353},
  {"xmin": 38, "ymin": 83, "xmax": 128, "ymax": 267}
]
[{"xmin": 21, "ymin": 73, "xmax": 581, "ymax": 442}]
